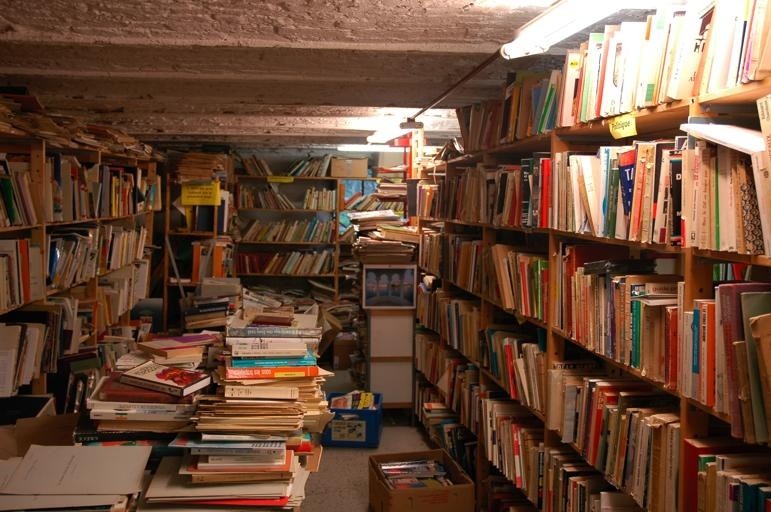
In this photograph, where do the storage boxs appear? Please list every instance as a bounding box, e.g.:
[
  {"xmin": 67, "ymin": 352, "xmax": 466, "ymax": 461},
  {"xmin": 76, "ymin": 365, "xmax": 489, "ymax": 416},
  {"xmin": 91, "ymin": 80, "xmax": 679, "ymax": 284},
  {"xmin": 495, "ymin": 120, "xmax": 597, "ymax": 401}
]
[{"xmin": 369, "ymin": 450, "xmax": 475, "ymax": 510}]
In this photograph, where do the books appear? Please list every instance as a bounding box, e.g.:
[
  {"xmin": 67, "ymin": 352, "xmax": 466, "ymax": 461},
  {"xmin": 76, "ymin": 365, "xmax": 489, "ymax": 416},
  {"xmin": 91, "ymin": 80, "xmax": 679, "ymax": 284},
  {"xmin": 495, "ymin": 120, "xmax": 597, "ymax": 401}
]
[{"xmin": 0, "ymin": 2, "xmax": 769, "ymax": 510}]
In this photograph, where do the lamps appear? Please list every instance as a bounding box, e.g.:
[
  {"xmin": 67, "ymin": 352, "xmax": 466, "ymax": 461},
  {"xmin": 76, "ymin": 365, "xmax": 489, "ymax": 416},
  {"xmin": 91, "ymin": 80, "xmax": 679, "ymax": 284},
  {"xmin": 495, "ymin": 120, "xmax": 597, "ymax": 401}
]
[{"xmin": 499, "ymin": 0, "xmax": 629, "ymax": 59}]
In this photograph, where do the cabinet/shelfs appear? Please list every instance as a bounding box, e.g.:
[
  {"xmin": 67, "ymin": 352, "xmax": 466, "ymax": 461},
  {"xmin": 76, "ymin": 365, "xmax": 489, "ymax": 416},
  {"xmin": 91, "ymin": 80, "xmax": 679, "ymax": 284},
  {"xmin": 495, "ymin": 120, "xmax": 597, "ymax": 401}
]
[
  {"xmin": 339, "ymin": 179, "xmax": 414, "ymax": 299},
  {"xmin": 167, "ymin": 172, "xmax": 232, "ymax": 329},
  {"xmin": 235, "ymin": 174, "xmax": 340, "ymax": 302},
  {"xmin": 418, "ymin": 77, "xmax": 769, "ymax": 511},
  {"xmin": 0, "ymin": 136, "xmax": 155, "ymax": 397}
]
